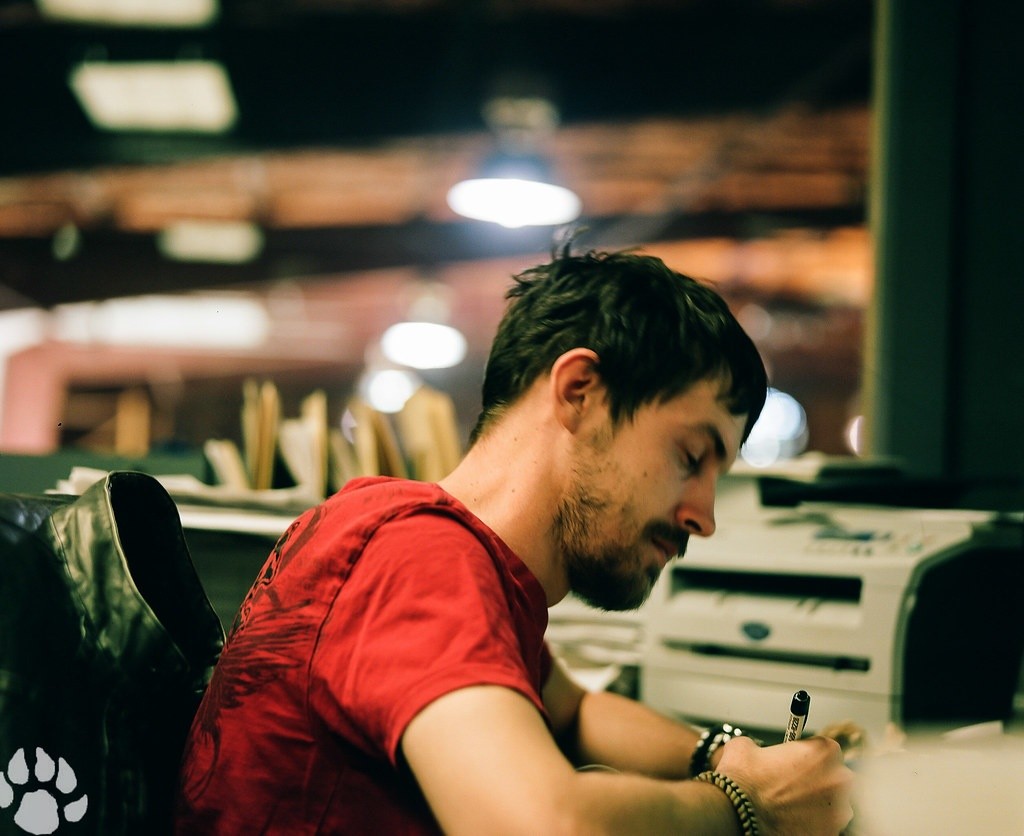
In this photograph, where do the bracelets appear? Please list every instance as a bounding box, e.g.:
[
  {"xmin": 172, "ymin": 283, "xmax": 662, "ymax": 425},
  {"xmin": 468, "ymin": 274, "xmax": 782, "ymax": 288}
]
[
  {"xmin": 690, "ymin": 723, "xmax": 748, "ymax": 779},
  {"xmin": 693, "ymin": 771, "xmax": 762, "ymax": 836}
]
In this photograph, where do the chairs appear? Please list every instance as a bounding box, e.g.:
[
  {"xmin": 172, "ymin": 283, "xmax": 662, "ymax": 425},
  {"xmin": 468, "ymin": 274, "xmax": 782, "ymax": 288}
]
[{"xmin": 1, "ymin": 467, "xmax": 215, "ymax": 836}]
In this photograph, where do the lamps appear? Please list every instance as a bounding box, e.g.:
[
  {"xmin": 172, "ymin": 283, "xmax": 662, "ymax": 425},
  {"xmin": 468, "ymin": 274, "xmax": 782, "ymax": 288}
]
[{"xmin": 444, "ymin": 94, "xmax": 582, "ymax": 228}]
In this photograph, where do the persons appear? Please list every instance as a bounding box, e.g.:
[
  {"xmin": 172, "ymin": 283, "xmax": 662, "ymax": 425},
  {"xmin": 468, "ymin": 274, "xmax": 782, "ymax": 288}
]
[{"xmin": 173, "ymin": 226, "xmax": 859, "ymax": 836}]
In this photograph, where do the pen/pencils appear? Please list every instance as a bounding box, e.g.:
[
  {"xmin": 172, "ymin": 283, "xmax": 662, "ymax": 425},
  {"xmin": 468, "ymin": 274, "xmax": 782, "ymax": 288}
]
[{"xmin": 783, "ymin": 690, "xmax": 811, "ymax": 744}]
